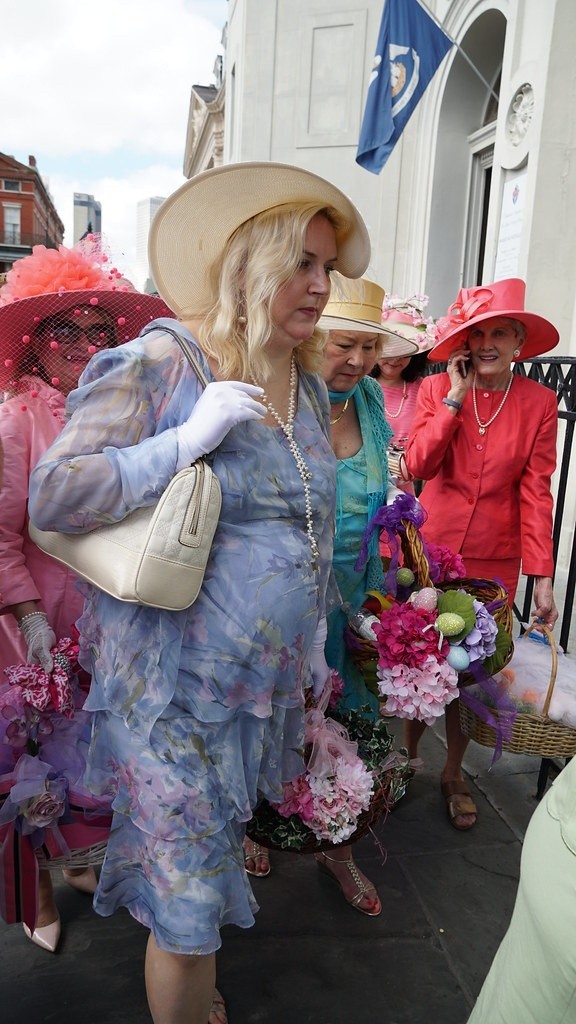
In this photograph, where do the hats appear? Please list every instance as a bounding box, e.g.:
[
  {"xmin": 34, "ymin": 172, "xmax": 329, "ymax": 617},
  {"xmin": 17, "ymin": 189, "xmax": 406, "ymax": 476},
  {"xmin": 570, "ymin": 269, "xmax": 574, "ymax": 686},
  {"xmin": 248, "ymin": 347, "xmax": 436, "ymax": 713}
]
[
  {"xmin": 427, "ymin": 279, "xmax": 559, "ymax": 363},
  {"xmin": 314, "ymin": 270, "xmax": 420, "ymax": 359},
  {"xmin": 381, "ymin": 296, "xmax": 434, "ymax": 357},
  {"xmin": 147, "ymin": 161, "xmax": 370, "ymax": 318},
  {"xmin": 0, "ymin": 245, "xmax": 176, "ymax": 391}
]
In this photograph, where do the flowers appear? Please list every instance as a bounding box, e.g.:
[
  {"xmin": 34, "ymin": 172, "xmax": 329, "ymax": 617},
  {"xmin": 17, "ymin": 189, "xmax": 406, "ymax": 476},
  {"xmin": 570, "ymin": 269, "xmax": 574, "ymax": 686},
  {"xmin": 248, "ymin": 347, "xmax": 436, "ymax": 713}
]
[
  {"xmin": 246, "ymin": 669, "xmax": 424, "ymax": 851},
  {"xmin": 381, "ymin": 294, "xmax": 438, "ymax": 349},
  {"xmin": 1, "ymin": 244, "xmax": 101, "ymax": 306},
  {"xmin": 347, "ymin": 544, "xmax": 512, "ymax": 722},
  {"xmin": 0, "ymin": 637, "xmax": 80, "ymax": 833}
]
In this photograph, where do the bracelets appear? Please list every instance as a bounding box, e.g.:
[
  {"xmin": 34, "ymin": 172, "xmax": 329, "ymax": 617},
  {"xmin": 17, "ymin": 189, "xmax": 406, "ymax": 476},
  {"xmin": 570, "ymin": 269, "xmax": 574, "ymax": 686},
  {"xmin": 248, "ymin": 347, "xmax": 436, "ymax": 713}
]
[{"xmin": 442, "ymin": 398, "xmax": 462, "ymax": 409}]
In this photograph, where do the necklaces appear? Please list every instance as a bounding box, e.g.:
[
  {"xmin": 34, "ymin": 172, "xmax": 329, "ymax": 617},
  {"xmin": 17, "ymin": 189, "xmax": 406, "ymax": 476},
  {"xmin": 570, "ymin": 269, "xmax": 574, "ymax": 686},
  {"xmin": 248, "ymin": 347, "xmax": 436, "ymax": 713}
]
[
  {"xmin": 385, "ymin": 380, "xmax": 406, "ymax": 417},
  {"xmin": 330, "ymin": 399, "xmax": 349, "ymax": 425},
  {"xmin": 472, "ymin": 370, "xmax": 514, "ymax": 428},
  {"xmin": 250, "ymin": 357, "xmax": 319, "ymax": 558}
]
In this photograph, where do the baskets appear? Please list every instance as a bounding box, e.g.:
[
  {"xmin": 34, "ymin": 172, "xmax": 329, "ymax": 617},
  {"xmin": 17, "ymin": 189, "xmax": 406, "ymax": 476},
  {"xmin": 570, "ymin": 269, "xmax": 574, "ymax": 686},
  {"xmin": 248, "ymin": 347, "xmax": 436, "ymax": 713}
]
[
  {"xmin": 31, "ymin": 710, "xmax": 108, "ymax": 869},
  {"xmin": 348, "ymin": 505, "xmax": 515, "ymax": 699},
  {"xmin": 244, "ymin": 695, "xmax": 390, "ymax": 853}
]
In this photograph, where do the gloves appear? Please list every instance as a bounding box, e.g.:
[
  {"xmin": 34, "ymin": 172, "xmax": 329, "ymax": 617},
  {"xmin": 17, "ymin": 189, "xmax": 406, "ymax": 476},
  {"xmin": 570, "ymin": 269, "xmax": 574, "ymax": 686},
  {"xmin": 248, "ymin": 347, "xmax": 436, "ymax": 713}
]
[
  {"xmin": 157, "ymin": 381, "xmax": 267, "ymax": 473},
  {"xmin": 307, "ymin": 617, "xmax": 331, "ymax": 696},
  {"xmin": 386, "ymin": 481, "xmax": 420, "ymax": 518},
  {"xmin": 19, "ymin": 616, "xmax": 57, "ymax": 674}
]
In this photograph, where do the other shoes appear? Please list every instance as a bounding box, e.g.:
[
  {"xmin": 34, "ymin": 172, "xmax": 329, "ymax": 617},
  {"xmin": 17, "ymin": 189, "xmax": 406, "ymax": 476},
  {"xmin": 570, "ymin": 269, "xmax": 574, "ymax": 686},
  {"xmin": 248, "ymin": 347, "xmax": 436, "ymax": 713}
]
[
  {"xmin": 23, "ymin": 909, "xmax": 62, "ymax": 952},
  {"xmin": 63, "ymin": 867, "xmax": 98, "ymax": 893}
]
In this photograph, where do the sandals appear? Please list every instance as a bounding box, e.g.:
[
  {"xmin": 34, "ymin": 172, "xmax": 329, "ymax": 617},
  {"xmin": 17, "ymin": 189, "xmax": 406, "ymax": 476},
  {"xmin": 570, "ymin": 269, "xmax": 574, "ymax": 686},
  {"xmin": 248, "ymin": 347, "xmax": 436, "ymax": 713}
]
[
  {"xmin": 244, "ymin": 840, "xmax": 270, "ymax": 877},
  {"xmin": 315, "ymin": 851, "xmax": 382, "ymax": 916}
]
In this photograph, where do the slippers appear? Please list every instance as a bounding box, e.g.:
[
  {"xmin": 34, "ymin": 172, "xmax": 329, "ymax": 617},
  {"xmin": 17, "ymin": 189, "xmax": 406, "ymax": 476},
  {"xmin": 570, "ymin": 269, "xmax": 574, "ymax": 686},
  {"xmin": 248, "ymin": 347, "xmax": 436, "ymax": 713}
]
[{"xmin": 441, "ymin": 778, "xmax": 476, "ymax": 830}]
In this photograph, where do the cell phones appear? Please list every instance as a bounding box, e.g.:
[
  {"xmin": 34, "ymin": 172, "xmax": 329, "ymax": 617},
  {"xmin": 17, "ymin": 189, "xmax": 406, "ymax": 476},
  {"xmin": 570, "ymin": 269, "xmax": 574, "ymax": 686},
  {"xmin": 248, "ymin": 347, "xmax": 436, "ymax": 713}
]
[{"xmin": 460, "ymin": 352, "xmax": 471, "ymax": 378}]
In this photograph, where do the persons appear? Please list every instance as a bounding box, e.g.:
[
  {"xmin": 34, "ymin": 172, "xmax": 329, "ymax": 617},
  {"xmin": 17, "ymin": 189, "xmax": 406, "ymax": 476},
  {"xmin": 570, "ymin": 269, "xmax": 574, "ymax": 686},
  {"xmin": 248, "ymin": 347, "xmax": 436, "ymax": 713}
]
[
  {"xmin": 243, "ymin": 271, "xmax": 419, "ymax": 915},
  {"xmin": 0, "ymin": 231, "xmax": 176, "ymax": 951},
  {"xmin": 29, "ymin": 161, "xmax": 371, "ymax": 1024},
  {"xmin": 369, "ymin": 293, "xmax": 437, "ymax": 496},
  {"xmin": 403, "ymin": 278, "xmax": 559, "ymax": 830}
]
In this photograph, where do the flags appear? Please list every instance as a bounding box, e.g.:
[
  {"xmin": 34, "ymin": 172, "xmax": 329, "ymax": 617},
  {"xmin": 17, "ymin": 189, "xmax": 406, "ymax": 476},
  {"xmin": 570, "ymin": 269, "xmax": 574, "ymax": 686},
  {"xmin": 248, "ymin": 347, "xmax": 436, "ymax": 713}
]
[{"xmin": 355, "ymin": 0, "xmax": 455, "ymax": 175}]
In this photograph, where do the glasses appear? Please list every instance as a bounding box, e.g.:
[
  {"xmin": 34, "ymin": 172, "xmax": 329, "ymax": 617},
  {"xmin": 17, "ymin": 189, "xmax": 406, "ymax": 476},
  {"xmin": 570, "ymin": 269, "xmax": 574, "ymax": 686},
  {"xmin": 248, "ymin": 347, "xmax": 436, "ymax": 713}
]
[{"xmin": 44, "ymin": 318, "xmax": 117, "ymax": 347}]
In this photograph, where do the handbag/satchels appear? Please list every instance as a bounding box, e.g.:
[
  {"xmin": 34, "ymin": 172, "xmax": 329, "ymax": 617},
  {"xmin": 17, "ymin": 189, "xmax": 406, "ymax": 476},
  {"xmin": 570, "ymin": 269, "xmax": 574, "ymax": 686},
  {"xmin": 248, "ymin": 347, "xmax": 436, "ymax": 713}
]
[
  {"xmin": 458, "ymin": 621, "xmax": 576, "ymax": 759},
  {"xmin": 28, "ymin": 326, "xmax": 221, "ymax": 612}
]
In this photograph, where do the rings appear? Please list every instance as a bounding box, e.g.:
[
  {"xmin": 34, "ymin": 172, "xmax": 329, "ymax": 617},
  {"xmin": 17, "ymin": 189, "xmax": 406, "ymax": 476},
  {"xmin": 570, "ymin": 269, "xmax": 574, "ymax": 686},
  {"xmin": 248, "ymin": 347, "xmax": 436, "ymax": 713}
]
[{"xmin": 549, "ymin": 624, "xmax": 554, "ymax": 628}]
